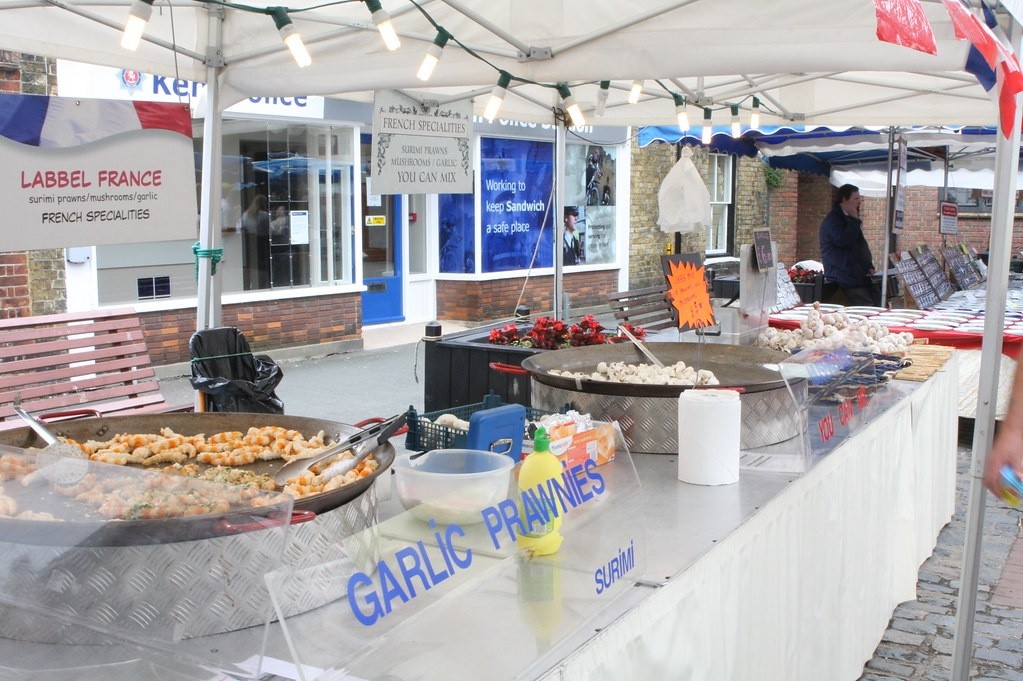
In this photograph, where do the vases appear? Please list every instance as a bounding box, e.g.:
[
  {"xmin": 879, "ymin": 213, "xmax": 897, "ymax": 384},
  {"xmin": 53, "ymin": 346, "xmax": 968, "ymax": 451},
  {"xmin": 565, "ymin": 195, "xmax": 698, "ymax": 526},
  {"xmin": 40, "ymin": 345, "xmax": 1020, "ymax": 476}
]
[{"xmin": 793, "ymin": 283, "xmax": 816, "ymax": 302}]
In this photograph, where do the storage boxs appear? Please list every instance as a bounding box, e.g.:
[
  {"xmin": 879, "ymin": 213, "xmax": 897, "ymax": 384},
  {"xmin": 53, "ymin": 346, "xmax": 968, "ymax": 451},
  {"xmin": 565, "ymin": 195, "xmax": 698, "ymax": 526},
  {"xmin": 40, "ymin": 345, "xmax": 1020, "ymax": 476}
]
[
  {"xmin": 873, "ymin": 273, "xmax": 898, "ymax": 300},
  {"xmin": 679, "ymin": 241, "xmax": 778, "ymax": 346},
  {"xmin": 405, "ymin": 389, "xmax": 574, "ymax": 454},
  {"xmin": 514, "ymin": 418, "xmax": 617, "ymax": 480}
]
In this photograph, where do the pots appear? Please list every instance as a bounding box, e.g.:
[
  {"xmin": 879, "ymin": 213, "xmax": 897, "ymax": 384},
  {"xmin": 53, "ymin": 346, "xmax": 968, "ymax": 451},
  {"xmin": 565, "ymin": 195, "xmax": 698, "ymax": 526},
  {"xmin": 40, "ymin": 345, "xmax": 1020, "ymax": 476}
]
[
  {"xmin": 0, "ymin": 409, "xmax": 396, "ymax": 546},
  {"xmin": 520, "ymin": 341, "xmax": 807, "ymax": 398}
]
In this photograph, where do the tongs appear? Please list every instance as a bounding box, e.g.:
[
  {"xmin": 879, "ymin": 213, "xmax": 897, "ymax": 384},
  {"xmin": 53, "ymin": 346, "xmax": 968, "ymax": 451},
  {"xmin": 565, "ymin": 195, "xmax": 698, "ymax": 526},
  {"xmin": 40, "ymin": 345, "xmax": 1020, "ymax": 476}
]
[{"xmin": 273, "ymin": 412, "xmax": 410, "ymax": 485}]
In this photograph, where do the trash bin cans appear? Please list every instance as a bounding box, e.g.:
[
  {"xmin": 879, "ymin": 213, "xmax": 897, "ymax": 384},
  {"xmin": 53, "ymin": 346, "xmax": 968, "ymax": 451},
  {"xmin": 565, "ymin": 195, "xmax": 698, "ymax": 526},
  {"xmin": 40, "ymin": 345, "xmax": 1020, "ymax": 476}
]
[{"xmin": 190, "ymin": 326, "xmax": 283, "ymax": 414}]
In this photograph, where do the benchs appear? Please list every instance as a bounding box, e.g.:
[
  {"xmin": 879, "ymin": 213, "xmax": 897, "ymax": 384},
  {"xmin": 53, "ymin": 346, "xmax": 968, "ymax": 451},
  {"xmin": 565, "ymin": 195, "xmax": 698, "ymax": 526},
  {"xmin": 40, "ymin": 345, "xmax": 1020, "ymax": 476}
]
[
  {"xmin": 0, "ymin": 305, "xmax": 195, "ymax": 428},
  {"xmin": 603, "ymin": 284, "xmax": 677, "ymax": 334}
]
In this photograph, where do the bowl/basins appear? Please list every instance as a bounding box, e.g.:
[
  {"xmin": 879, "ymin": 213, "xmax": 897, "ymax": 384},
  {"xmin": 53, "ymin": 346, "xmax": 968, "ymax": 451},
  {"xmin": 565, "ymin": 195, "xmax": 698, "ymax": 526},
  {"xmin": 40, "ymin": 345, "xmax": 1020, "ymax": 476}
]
[{"xmin": 391, "ymin": 449, "xmax": 516, "ymax": 524}]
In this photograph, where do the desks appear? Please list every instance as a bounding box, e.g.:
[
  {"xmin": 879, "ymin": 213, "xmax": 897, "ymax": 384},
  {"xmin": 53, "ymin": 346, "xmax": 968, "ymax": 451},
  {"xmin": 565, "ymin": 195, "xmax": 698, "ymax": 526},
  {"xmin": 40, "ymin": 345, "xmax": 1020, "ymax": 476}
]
[
  {"xmin": 768, "ymin": 271, "xmax": 1023, "ymax": 448},
  {"xmin": 0, "ymin": 350, "xmax": 959, "ymax": 681}
]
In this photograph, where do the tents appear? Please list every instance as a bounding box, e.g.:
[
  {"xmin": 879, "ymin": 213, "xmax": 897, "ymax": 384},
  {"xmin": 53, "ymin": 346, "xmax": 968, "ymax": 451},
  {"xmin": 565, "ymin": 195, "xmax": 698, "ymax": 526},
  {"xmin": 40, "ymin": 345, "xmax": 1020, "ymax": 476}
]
[{"xmin": 0, "ymin": 0, "xmax": 1023, "ymax": 681}]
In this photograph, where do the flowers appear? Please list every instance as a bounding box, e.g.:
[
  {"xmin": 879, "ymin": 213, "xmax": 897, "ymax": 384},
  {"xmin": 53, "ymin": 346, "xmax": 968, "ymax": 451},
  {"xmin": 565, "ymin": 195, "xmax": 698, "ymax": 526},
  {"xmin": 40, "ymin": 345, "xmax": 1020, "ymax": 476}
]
[{"xmin": 788, "ymin": 265, "xmax": 823, "ymax": 283}]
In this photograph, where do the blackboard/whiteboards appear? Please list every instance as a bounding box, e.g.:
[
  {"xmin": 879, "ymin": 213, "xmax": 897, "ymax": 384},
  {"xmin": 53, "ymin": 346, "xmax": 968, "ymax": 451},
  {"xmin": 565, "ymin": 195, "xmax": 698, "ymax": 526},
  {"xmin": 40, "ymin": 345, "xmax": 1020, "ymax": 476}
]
[
  {"xmin": 370, "ymin": 88, "xmax": 473, "ymax": 195},
  {"xmin": 0, "ymin": 93, "xmax": 198, "ymax": 252}
]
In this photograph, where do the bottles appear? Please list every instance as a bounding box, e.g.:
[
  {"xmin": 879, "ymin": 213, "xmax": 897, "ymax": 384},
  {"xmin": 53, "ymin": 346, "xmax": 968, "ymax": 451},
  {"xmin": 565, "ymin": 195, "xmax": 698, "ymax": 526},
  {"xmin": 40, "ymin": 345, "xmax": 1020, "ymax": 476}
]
[{"xmin": 513, "ymin": 425, "xmax": 564, "ymax": 556}]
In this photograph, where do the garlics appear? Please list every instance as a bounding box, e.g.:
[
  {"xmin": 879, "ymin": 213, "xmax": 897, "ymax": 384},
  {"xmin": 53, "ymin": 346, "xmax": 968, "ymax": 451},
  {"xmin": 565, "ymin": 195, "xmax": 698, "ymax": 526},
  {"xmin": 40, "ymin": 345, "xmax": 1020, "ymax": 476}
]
[{"xmin": 752, "ymin": 310, "xmax": 913, "ymax": 355}]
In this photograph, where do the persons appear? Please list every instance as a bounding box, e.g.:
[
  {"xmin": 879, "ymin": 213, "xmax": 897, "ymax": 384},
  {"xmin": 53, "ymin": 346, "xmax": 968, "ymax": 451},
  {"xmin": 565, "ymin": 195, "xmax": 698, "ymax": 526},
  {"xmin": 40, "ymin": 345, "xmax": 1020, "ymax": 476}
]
[
  {"xmin": 563, "ymin": 206, "xmax": 581, "ymax": 265},
  {"xmin": 982, "ymin": 348, "xmax": 1023, "ymax": 509},
  {"xmin": 240, "ymin": 194, "xmax": 291, "ymax": 237},
  {"xmin": 819, "ymin": 183, "xmax": 880, "ymax": 308}
]
